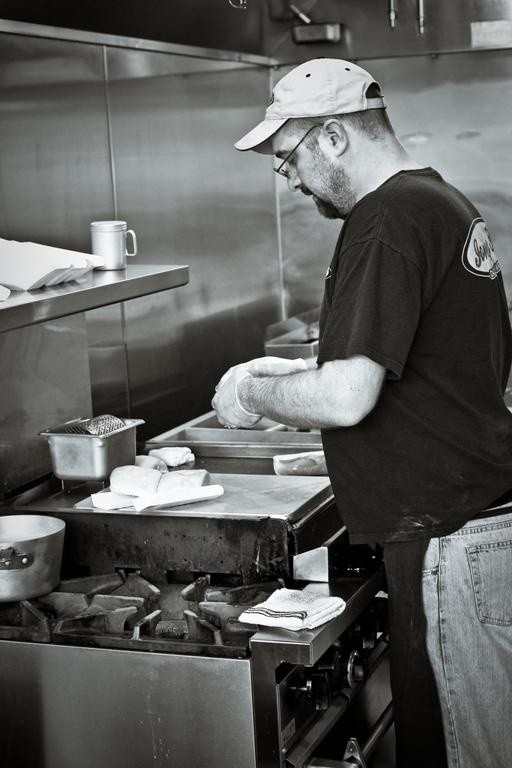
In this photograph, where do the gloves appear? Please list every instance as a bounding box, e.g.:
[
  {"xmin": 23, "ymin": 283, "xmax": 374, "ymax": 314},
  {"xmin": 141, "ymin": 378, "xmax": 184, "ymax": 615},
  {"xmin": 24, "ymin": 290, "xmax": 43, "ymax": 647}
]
[
  {"xmin": 214, "ymin": 356, "xmax": 308, "ymax": 394},
  {"xmin": 211, "ymin": 376, "xmax": 262, "ymax": 428}
]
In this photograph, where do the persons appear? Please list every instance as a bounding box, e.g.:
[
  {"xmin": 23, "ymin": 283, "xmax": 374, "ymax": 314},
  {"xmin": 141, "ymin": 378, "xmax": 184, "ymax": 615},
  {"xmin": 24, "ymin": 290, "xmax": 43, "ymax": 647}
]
[{"xmin": 211, "ymin": 55, "xmax": 512, "ymax": 765}]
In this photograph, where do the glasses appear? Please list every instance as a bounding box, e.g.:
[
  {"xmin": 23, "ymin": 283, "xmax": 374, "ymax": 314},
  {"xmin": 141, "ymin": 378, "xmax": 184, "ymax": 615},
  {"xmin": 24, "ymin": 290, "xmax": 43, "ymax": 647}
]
[{"xmin": 274, "ymin": 125, "xmax": 326, "ymax": 179}]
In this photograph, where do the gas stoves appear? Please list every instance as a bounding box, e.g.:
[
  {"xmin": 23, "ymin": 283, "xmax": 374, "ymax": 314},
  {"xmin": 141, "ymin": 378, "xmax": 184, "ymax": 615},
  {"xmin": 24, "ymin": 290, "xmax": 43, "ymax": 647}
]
[{"xmin": 0, "ymin": 566, "xmax": 289, "ymax": 656}]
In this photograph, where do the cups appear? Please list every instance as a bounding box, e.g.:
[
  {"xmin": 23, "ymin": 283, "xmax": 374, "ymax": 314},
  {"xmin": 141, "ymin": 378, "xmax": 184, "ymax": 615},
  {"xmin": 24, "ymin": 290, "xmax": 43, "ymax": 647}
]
[{"xmin": 89, "ymin": 218, "xmax": 139, "ymax": 272}]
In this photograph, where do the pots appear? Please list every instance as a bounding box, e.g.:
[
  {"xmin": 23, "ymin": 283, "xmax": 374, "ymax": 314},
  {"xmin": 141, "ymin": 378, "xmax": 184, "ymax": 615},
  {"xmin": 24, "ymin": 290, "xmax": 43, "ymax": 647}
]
[{"xmin": 0, "ymin": 513, "xmax": 68, "ymax": 603}]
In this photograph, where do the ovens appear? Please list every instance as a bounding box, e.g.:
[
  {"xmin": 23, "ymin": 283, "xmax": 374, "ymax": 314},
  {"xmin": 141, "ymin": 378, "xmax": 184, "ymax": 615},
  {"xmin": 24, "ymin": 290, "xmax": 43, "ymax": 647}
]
[{"xmin": 271, "ymin": 564, "xmax": 397, "ymax": 768}]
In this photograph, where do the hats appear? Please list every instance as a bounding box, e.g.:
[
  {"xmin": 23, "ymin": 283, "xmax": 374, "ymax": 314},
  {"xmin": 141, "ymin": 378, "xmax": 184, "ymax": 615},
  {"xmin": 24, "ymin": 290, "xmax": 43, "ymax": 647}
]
[{"xmin": 234, "ymin": 59, "xmax": 386, "ymax": 155}]
